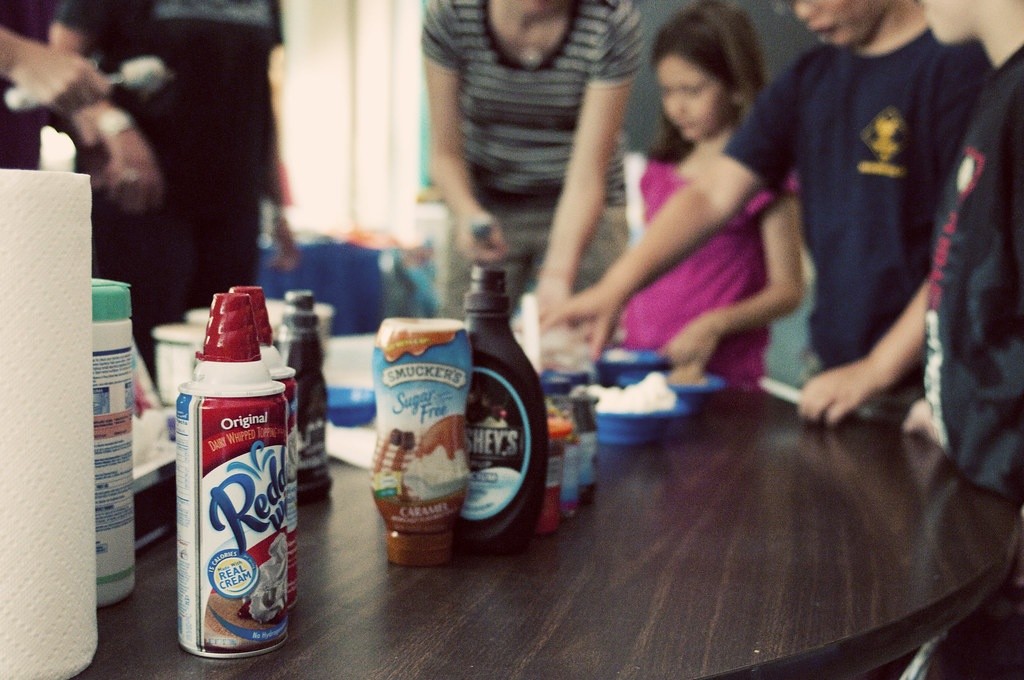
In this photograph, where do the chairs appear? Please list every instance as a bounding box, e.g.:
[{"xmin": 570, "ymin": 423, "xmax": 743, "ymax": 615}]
[{"xmin": 259, "ymin": 241, "xmax": 390, "ymax": 429}]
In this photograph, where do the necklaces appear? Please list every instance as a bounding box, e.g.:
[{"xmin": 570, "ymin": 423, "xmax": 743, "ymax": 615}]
[{"xmin": 519, "ymin": 47, "xmax": 544, "ymax": 72}]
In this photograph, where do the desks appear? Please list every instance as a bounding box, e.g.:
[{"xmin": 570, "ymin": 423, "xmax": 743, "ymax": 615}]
[{"xmin": 69, "ymin": 385, "xmax": 1017, "ymax": 680}]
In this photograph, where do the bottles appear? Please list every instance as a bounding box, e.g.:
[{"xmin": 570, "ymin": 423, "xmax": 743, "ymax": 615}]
[
  {"xmin": 278, "ymin": 290, "xmax": 333, "ymax": 500},
  {"xmin": 92, "ymin": 278, "xmax": 137, "ymax": 603},
  {"xmin": 175, "ymin": 292, "xmax": 289, "ymax": 658},
  {"xmin": 230, "ymin": 286, "xmax": 298, "ymax": 610},
  {"xmin": 369, "ymin": 318, "xmax": 473, "ymax": 565},
  {"xmin": 454, "ymin": 265, "xmax": 549, "ymax": 550}
]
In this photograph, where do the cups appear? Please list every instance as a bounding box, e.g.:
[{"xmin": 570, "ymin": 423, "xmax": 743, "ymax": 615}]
[{"xmin": 154, "ymin": 300, "xmax": 334, "ymax": 407}]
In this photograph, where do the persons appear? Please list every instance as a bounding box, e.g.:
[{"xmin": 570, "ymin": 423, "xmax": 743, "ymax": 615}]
[
  {"xmin": 508, "ymin": 0, "xmax": 985, "ymax": 429},
  {"xmin": 904, "ymin": 0, "xmax": 1024, "ymax": 510},
  {"xmin": 0, "ymin": 0, "xmax": 293, "ymax": 389},
  {"xmin": 573, "ymin": 0, "xmax": 808, "ymax": 396},
  {"xmin": 418, "ymin": 0, "xmax": 646, "ymax": 332}
]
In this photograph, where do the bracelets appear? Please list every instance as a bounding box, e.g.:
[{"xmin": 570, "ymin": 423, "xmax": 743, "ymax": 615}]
[{"xmin": 98, "ymin": 107, "xmax": 135, "ymax": 137}]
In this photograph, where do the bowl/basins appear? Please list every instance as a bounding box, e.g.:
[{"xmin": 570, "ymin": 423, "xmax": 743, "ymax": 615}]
[{"xmin": 595, "ymin": 342, "xmax": 721, "ymax": 445}]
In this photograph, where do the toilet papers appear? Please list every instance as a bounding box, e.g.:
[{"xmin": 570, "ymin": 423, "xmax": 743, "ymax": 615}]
[{"xmin": 0, "ymin": 168, "xmax": 99, "ymax": 675}]
